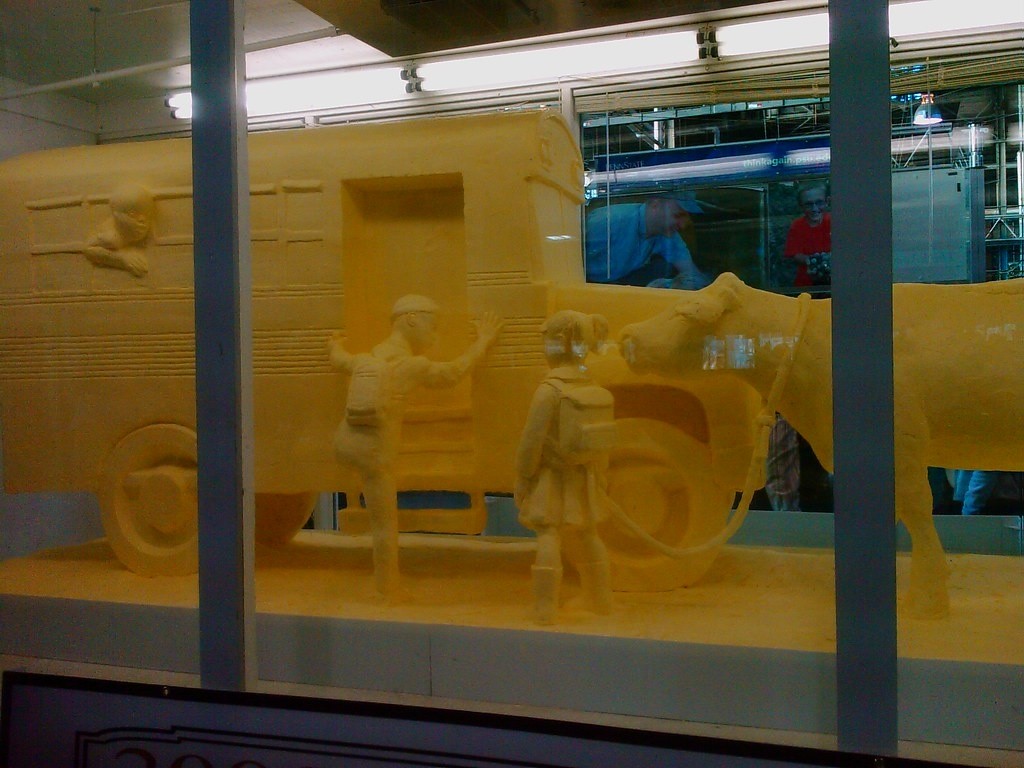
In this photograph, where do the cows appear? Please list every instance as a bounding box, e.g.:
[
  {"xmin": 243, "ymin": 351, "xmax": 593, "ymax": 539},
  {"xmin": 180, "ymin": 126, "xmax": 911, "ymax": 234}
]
[{"xmin": 614, "ymin": 272, "xmax": 1024, "ymax": 623}]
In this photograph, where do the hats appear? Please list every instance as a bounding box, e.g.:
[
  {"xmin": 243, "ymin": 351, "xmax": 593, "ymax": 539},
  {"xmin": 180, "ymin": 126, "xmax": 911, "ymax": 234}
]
[{"xmin": 647, "ymin": 181, "xmax": 705, "ymax": 214}]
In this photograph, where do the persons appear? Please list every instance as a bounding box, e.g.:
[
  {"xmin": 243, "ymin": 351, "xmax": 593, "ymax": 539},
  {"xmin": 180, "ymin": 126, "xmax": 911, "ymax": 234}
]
[
  {"xmin": 329, "ymin": 292, "xmax": 502, "ymax": 599},
  {"xmin": 761, "ymin": 410, "xmax": 997, "ymax": 514},
  {"xmin": 585, "ymin": 187, "xmax": 710, "ymax": 292},
  {"xmin": 784, "ymin": 180, "xmax": 831, "ymax": 288},
  {"xmin": 515, "ymin": 308, "xmax": 615, "ymax": 624}
]
